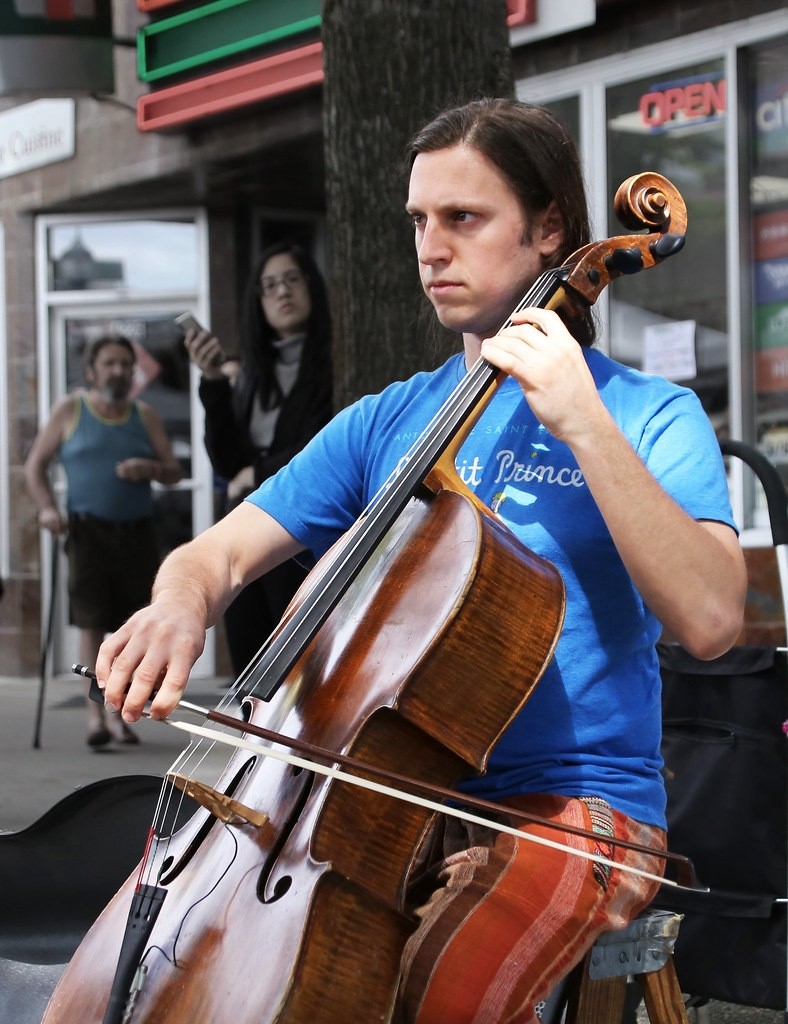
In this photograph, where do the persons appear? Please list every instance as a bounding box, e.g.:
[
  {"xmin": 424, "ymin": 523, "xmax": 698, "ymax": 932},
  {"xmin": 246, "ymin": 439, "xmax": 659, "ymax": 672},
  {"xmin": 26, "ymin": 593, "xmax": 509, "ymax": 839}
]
[
  {"xmin": 218, "ymin": 356, "xmax": 246, "ymax": 386},
  {"xmin": 27, "ymin": 334, "xmax": 183, "ymax": 747},
  {"xmin": 95, "ymin": 100, "xmax": 747, "ymax": 1024},
  {"xmin": 185, "ymin": 244, "xmax": 334, "ymax": 720}
]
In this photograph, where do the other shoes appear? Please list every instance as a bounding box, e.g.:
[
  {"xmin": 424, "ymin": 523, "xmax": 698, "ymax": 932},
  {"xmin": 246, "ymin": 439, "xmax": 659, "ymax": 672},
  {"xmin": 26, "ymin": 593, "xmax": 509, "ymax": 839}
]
[
  {"xmin": 88, "ymin": 724, "xmax": 112, "ymax": 752},
  {"xmin": 108, "ymin": 720, "xmax": 136, "ymax": 744}
]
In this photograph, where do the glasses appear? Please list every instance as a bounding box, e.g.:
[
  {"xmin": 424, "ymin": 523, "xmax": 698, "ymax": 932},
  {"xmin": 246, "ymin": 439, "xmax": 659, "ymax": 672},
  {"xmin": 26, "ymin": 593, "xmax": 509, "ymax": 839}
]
[{"xmin": 255, "ymin": 269, "xmax": 306, "ymax": 296}]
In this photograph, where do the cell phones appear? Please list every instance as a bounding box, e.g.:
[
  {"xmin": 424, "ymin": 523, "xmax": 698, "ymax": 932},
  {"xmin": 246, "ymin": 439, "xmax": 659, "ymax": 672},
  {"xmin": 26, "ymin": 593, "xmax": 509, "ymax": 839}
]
[{"xmin": 174, "ymin": 311, "xmax": 227, "ymax": 366}]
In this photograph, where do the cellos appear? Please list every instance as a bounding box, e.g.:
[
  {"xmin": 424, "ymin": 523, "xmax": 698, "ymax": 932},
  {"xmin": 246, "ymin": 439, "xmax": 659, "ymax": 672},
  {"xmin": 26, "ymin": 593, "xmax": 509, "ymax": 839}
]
[{"xmin": 32, "ymin": 172, "xmax": 688, "ymax": 1024}]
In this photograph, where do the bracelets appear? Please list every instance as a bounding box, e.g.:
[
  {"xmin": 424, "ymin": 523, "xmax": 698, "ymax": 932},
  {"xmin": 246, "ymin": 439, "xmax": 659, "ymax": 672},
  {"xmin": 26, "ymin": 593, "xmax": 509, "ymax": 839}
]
[{"xmin": 153, "ymin": 460, "xmax": 162, "ymax": 480}]
